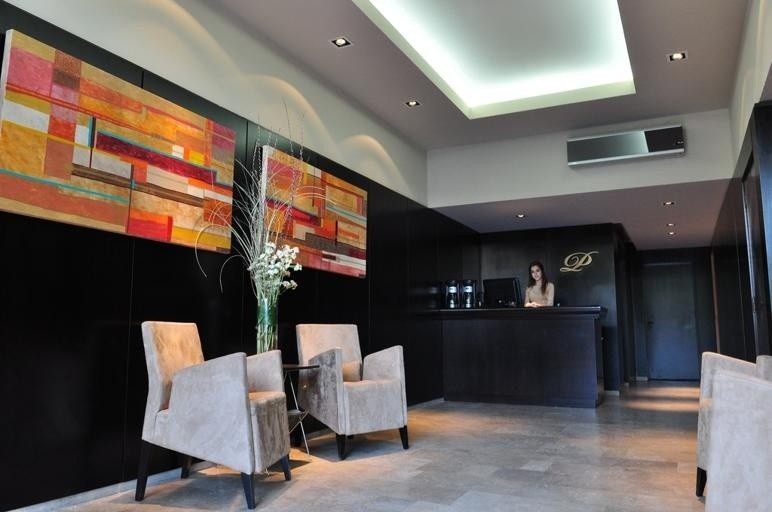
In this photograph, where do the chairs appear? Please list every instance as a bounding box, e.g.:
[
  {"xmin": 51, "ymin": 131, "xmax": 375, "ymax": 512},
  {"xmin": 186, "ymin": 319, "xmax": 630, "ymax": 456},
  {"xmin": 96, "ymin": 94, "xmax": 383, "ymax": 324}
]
[
  {"xmin": 694, "ymin": 351, "xmax": 772, "ymax": 511},
  {"xmin": 135, "ymin": 320, "xmax": 291, "ymax": 509},
  {"xmin": 293, "ymin": 323, "xmax": 409, "ymax": 460}
]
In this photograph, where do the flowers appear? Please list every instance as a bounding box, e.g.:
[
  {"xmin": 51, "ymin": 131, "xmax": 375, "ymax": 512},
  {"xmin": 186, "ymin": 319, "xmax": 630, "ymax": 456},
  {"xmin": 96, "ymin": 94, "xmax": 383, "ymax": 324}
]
[{"xmin": 194, "ymin": 100, "xmax": 335, "ymax": 298}]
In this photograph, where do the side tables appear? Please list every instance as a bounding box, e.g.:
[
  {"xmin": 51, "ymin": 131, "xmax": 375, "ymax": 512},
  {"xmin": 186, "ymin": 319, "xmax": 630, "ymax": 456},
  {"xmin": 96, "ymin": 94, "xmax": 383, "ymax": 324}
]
[{"xmin": 283, "ymin": 363, "xmax": 319, "ymax": 457}]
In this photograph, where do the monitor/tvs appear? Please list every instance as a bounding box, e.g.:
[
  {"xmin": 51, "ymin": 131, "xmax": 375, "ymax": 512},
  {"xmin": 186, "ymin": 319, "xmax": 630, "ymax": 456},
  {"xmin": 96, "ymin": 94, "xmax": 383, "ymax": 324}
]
[{"xmin": 482, "ymin": 278, "xmax": 524, "ymax": 306}]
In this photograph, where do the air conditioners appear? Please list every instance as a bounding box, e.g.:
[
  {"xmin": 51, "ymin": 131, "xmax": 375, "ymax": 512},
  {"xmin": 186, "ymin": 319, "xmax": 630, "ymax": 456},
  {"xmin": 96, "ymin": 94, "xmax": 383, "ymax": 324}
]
[{"xmin": 567, "ymin": 124, "xmax": 686, "ymax": 166}]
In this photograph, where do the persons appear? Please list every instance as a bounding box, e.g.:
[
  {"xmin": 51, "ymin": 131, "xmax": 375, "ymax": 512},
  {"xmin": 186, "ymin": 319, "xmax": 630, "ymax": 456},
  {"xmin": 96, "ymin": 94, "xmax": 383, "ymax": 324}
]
[{"xmin": 523, "ymin": 261, "xmax": 555, "ymax": 308}]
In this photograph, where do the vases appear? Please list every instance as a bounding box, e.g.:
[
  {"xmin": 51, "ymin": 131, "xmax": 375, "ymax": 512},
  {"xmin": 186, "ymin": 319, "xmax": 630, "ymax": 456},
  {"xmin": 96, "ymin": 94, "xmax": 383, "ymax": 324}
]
[{"xmin": 256, "ymin": 298, "xmax": 278, "ymax": 353}]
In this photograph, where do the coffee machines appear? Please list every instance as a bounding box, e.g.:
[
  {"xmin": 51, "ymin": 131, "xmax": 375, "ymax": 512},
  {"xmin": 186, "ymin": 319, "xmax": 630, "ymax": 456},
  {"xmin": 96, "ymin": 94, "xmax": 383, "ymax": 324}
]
[
  {"xmin": 459, "ymin": 279, "xmax": 477, "ymax": 309},
  {"xmin": 445, "ymin": 279, "xmax": 459, "ymax": 309}
]
